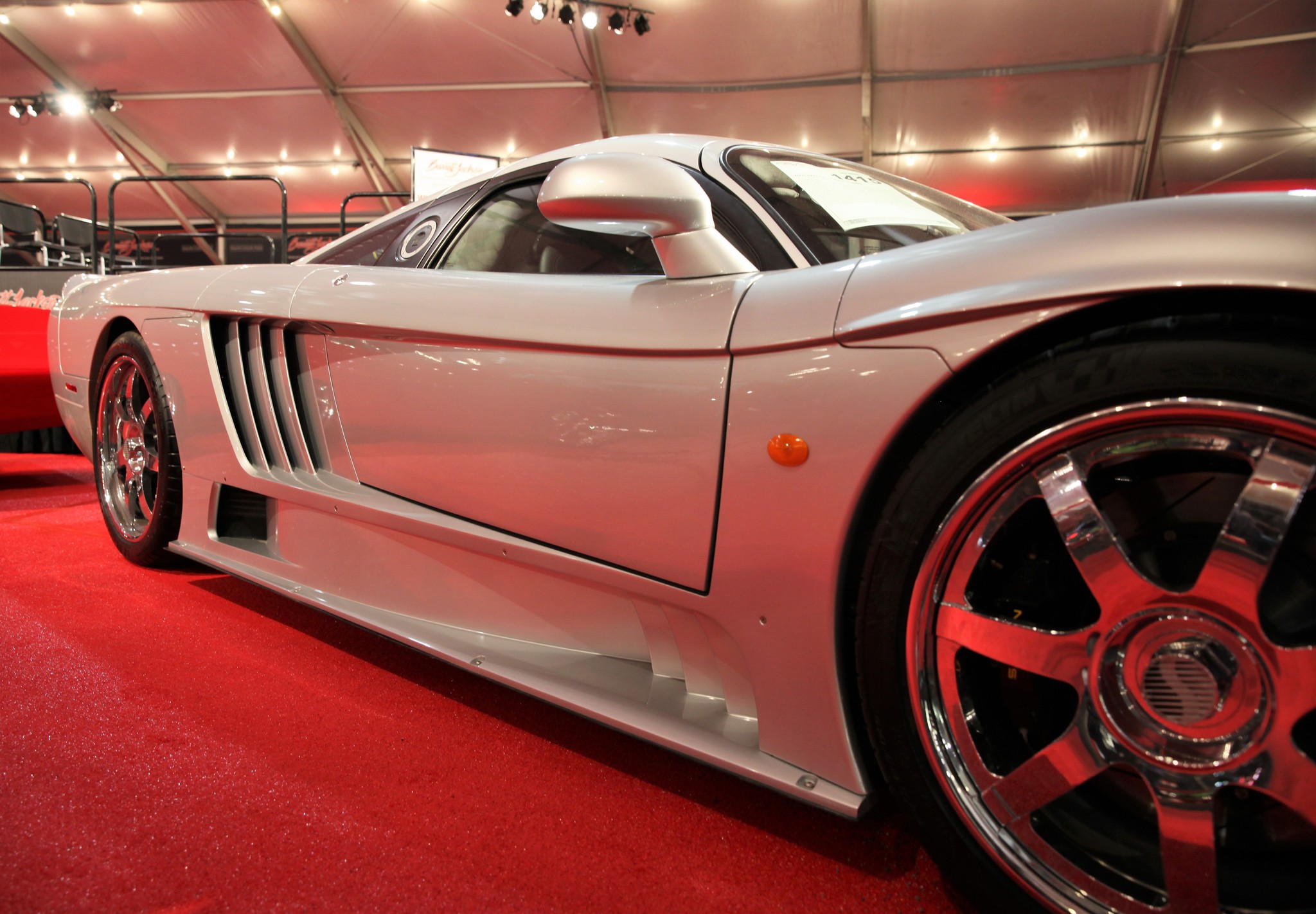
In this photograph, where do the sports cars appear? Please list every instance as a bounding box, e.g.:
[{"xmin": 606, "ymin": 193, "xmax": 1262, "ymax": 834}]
[
  {"xmin": 0, "ymin": 196, "xmax": 308, "ymax": 460},
  {"xmin": 47, "ymin": 131, "xmax": 1313, "ymax": 893}
]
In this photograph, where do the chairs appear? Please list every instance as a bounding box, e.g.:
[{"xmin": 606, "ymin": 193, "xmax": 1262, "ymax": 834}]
[{"xmin": 0, "ymin": 199, "xmax": 141, "ymax": 269}]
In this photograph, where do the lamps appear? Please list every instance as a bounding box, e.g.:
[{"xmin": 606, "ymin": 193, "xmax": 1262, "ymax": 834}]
[
  {"xmin": 557, "ymin": 2, "xmax": 575, "ymax": 24},
  {"xmin": 633, "ymin": 12, "xmax": 650, "ymax": 36},
  {"xmin": 504, "ymin": 0, "xmax": 523, "ymax": 17},
  {"xmin": 608, "ymin": 12, "xmax": 625, "ymax": 34},
  {"xmin": 530, "ymin": 0, "xmax": 547, "ymax": 20},
  {"xmin": 8, "ymin": 93, "xmax": 126, "ymax": 119}
]
